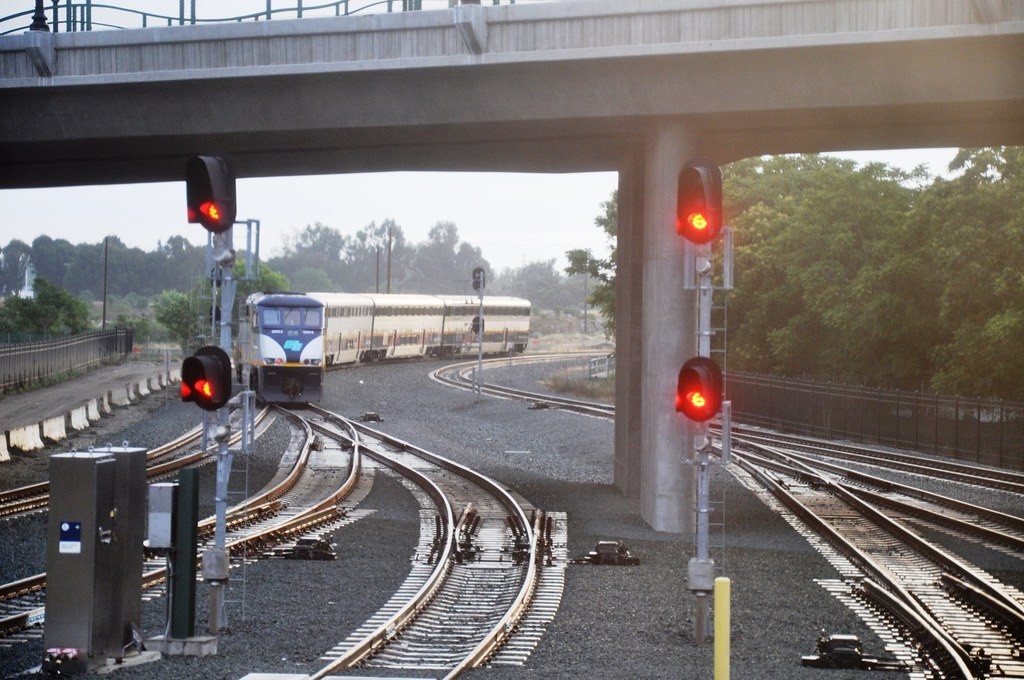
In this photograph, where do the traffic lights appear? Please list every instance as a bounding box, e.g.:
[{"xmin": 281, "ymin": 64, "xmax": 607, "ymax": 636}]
[
  {"xmin": 675, "ymin": 356, "xmax": 728, "ymax": 426},
  {"xmin": 185, "ymin": 152, "xmax": 237, "ymax": 235},
  {"xmin": 177, "ymin": 347, "xmax": 235, "ymax": 411},
  {"xmin": 677, "ymin": 157, "xmax": 724, "ymax": 245}
]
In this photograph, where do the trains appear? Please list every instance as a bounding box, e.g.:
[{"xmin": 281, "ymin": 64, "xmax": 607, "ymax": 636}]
[{"xmin": 244, "ymin": 293, "xmax": 532, "ymax": 405}]
